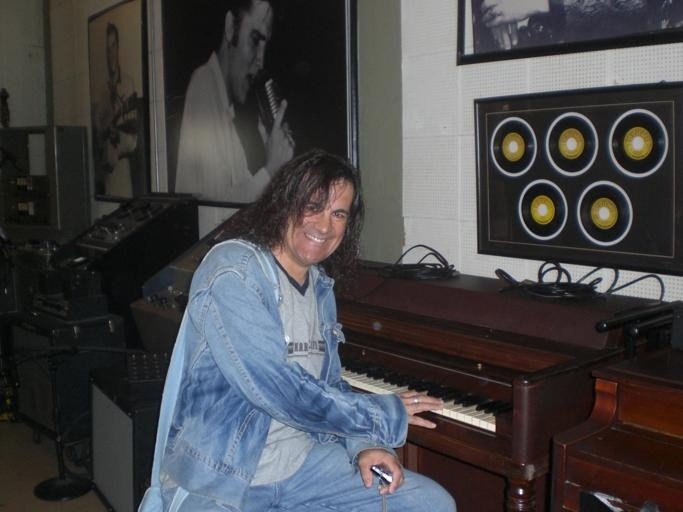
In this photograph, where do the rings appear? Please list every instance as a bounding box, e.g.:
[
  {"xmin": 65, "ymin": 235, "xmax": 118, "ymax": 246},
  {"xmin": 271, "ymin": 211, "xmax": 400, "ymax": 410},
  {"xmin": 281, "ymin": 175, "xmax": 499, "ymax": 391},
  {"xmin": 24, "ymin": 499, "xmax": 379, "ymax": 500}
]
[{"xmin": 412, "ymin": 395, "xmax": 420, "ymax": 404}]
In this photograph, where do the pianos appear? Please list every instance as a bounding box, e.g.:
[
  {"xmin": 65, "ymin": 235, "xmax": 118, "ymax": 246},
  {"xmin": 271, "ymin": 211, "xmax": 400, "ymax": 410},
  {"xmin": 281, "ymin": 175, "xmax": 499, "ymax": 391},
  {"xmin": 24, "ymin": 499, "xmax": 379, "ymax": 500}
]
[
  {"xmin": 548, "ymin": 314, "xmax": 682, "ymax": 511},
  {"xmin": 331, "ymin": 257, "xmax": 679, "ymax": 511}
]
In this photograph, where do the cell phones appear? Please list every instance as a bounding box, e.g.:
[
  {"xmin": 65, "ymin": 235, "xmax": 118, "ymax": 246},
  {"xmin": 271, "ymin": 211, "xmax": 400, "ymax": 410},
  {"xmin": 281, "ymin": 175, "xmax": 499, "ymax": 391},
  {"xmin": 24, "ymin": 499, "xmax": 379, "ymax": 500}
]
[{"xmin": 370, "ymin": 465, "xmax": 393, "ymax": 484}]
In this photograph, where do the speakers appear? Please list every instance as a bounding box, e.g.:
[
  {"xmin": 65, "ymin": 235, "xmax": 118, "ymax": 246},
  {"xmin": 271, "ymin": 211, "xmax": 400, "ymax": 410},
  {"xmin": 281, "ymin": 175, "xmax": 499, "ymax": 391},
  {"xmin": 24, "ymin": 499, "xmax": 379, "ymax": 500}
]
[
  {"xmin": 90, "ymin": 377, "xmax": 164, "ymax": 512},
  {"xmin": 11, "ymin": 315, "xmax": 124, "ymax": 441}
]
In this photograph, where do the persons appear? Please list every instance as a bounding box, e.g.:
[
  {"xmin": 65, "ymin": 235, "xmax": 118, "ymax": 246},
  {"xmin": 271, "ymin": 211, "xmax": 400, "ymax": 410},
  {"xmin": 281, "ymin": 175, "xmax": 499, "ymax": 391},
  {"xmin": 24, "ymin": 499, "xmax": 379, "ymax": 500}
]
[
  {"xmin": 90, "ymin": 22, "xmax": 143, "ymax": 198},
  {"xmin": 479, "ymin": 0, "xmax": 683, "ymax": 51},
  {"xmin": 174, "ymin": 0, "xmax": 295, "ymax": 203},
  {"xmin": 136, "ymin": 147, "xmax": 457, "ymax": 512}
]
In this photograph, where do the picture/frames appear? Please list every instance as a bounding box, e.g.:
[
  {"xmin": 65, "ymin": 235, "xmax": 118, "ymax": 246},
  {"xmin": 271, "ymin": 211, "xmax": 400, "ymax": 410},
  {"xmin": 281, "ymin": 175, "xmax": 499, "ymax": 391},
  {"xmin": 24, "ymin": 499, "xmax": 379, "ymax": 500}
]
[
  {"xmin": 88, "ymin": 0, "xmax": 153, "ymax": 202},
  {"xmin": 161, "ymin": 0, "xmax": 359, "ymax": 210},
  {"xmin": 456, "ymin": 0, "xmax": 683, "ymax": 66}
]
